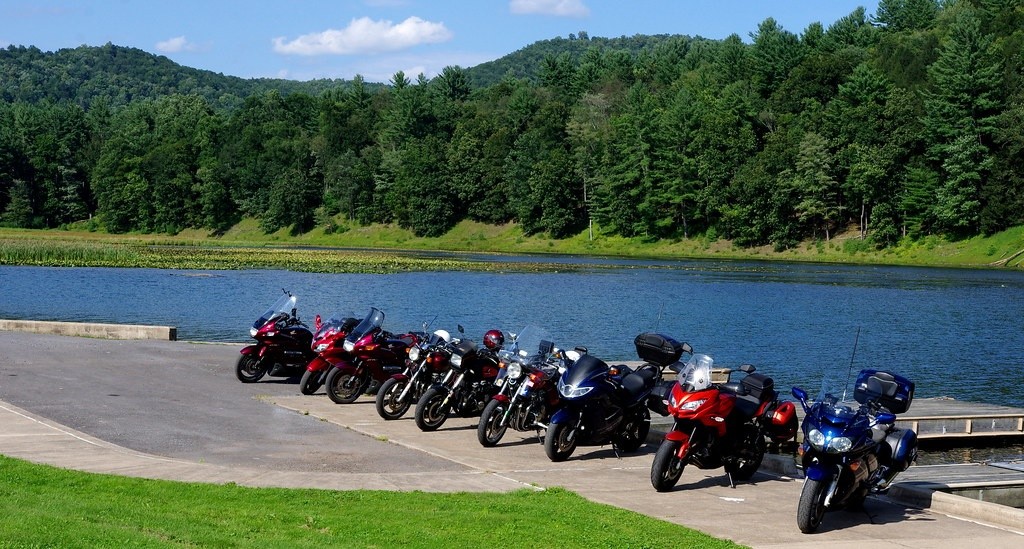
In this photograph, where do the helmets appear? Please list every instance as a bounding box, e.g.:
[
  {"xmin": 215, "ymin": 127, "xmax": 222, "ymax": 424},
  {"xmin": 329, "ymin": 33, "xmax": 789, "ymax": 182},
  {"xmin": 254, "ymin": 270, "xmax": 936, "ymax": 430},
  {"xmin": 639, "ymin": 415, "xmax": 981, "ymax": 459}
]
[
  {"xmin": 484, "ymin": 329, "xmax": 504, "ymax": 351},
  {"xmin": 435, "ymin": 329, "xmax": 449, "ymax": 344}
]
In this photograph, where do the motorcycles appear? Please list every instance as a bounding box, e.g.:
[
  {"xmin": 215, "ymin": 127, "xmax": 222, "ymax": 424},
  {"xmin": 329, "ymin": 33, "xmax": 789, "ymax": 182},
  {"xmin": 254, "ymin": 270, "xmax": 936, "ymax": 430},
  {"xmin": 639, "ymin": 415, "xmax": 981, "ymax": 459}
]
[
  {"xmin": 235, "ymin": 285, "xmax": 423, "ymax": 405},
  {"xmin": 542, "ymin": 328, "xmax": 687, "ymax": 463},
  {"xmin": 651, "ymin": 341, "xmax": 800, "ymax": 493},
  {"xmin": 790, "ymin": 368, "xmax": 921, "ymax": 537},
  {"xmin": 474, "ymin": 324, "xmax": 587, "ymax": 447},
  {"xmin": 414, "ymin": 323, "xmax": 510, "ymax": 432},
  {"xmin": 375, "ymin": 320, "xmax": 465, "ymax": 422}
]
